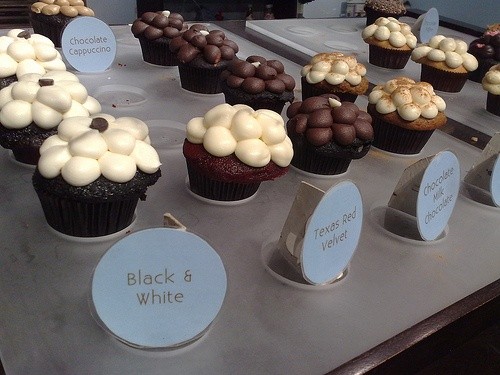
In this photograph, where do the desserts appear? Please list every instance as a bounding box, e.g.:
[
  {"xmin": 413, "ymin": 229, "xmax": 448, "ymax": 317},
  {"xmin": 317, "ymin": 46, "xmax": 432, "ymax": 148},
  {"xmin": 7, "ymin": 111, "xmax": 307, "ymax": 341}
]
[
  {"xmin": 30, "ymin": 0, "xmax": 95, "ymax": 48},
  {"xmin": 183, "ymin": 103, "xmax": 293, "ymax": 202},
  {"xmin": 367, "ymin": 77, "xmax": 448, "ymax": 155},
  {"xmin": 482, "ymin": 65, "xmax": 500, "ymax": 117},
  {"xmin": 364, "ymin": 0, "xmax": 407, "ymax": 25},
  {"xmin": 31, "ymin": 114, "xmax": 163, "ymax": 238},
  {"xmin": 0, "ymin": 29, "xmax": 101, "ymax": 166},
  {"xmin": 287, "ymin": 93, "xmax": 373, "ymax": 176},
  {"xmin": 467, "ymin": 23, "xmax": 500, "ymax": 83},
  {"xmin": 131, "ymin": 10, "xmax": 188, "ymax": 66},
  {"xmin": 169, "ymin": 24, "xmax": 238, "ymax": 94},
  {"xmin": 301, "ymin": 52, "xmax": 369, "ymax": 103},
  {"xmin": 362, "ymin": 17, "xmax": 417, "ymax": 69},
  {"xmin": 221, "ymin": 56, "xmax": 295, "ymax": 115},
  {"xmin": 411, "ymin": 34, "xmax": 479, "ymax": 93}
]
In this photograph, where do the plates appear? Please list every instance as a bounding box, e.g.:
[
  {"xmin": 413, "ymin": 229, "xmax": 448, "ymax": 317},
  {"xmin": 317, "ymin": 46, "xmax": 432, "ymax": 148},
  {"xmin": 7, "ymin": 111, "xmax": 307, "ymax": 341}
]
[
  {"xmin": 458, "ymin": 191, "xmax": 500, "ymax": 211},
  {"xmin": 288, "ymin": 164, "xmax": 351, "ymax": 180},
  {"xmin": 142, "ymin": 57, "xmax": 178, "ymax": 68},
  {"xmin": 259, "ymin": 230, "xmax": 352, "ymax": 291},
  {"xmin": 8, "ymin": 149, "xmax": 37, "ymax": 169},
  {"xmin": 325, "ymin": 23, "xmax": 360, "ymax": 34},
  {"xmin": 484, "ymin": 110, "xmax": 500, "ymax": 119},
  {"xmin": 287, "ymin": 25, "xmax": 319, "ymax": 37},
  {"xmin": 370, "ymin": 145, "xmax": 423, "ymax": 158},
  {"xmin": 368, "ymin": 62, "xmax": 405, "ymax": 72},
  {"xmin": 178, "ymin": 81, "xmax": 223, "ymax": 97},
  {"xmin": 143, "ymin": 119, "xmax": 187, "ymax": 149},
  {"xmin": 324, "ymin": 40, "xmax": 358, "ymax": 50},
  {"xmin": 86, "ymin": 271, "xmax": 223, "ymax": 352},
  {"xmin": 434, "ymin": 90, "xmax": 462, "ymax": 96},
  {"xmin": 40, "ymin": 210, "xmax": 138, "ymax": 244},
  {"xmin": 94, "ymin": 84, "xmax": 148, "ymax": 107},
  {"xmin": 184, "ymin": 175, "xmax": 263, "ymax": 205},
  {"xmin": 368, "ymin": 197, "xmax": 449, "ymax": 246}
]
